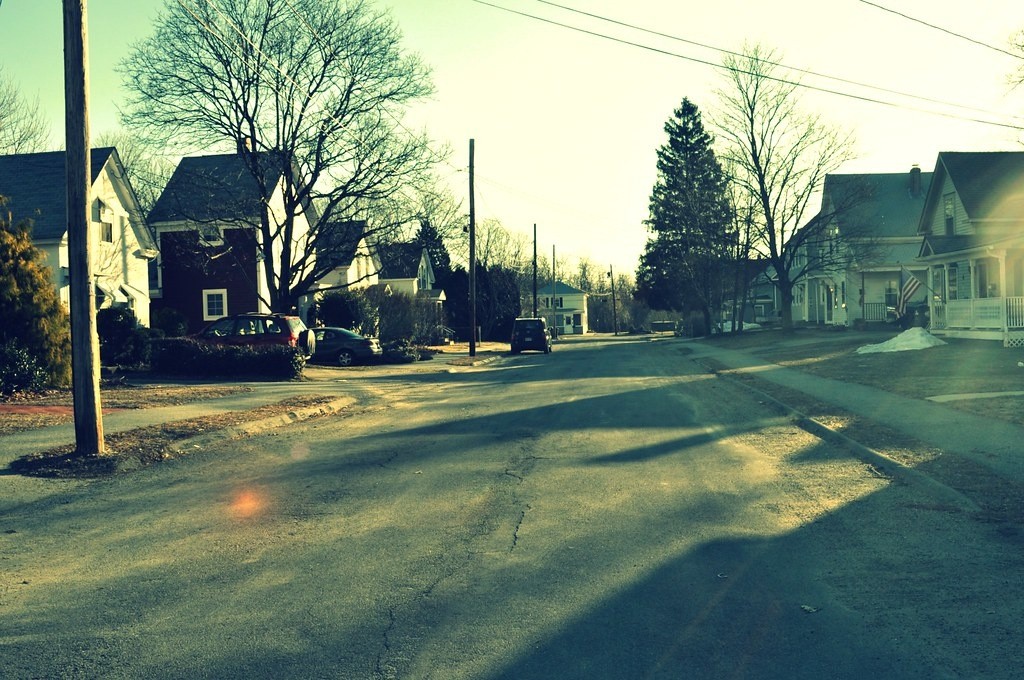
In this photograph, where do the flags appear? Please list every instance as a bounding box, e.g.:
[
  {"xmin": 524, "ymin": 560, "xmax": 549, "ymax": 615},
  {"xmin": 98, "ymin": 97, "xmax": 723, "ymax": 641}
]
[{"xmin": 898, "ymin": 267, "xmax": 919, "ymax": 317}]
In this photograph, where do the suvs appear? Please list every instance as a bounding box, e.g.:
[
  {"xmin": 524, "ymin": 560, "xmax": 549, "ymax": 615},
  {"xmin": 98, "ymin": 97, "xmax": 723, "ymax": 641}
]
[
  {"xmin": 510, "ymin": 318, "xmax": 553, "ymax": 354},
  {"xmin": 183, "ymin": 311, "xmax": 316, "ymax": 361}
]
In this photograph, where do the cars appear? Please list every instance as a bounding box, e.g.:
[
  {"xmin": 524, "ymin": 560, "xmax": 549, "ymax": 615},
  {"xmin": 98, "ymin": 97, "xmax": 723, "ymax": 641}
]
[{"xmin": 308, "ymin": 327, "xmax": 383, "ymax": 366}]
